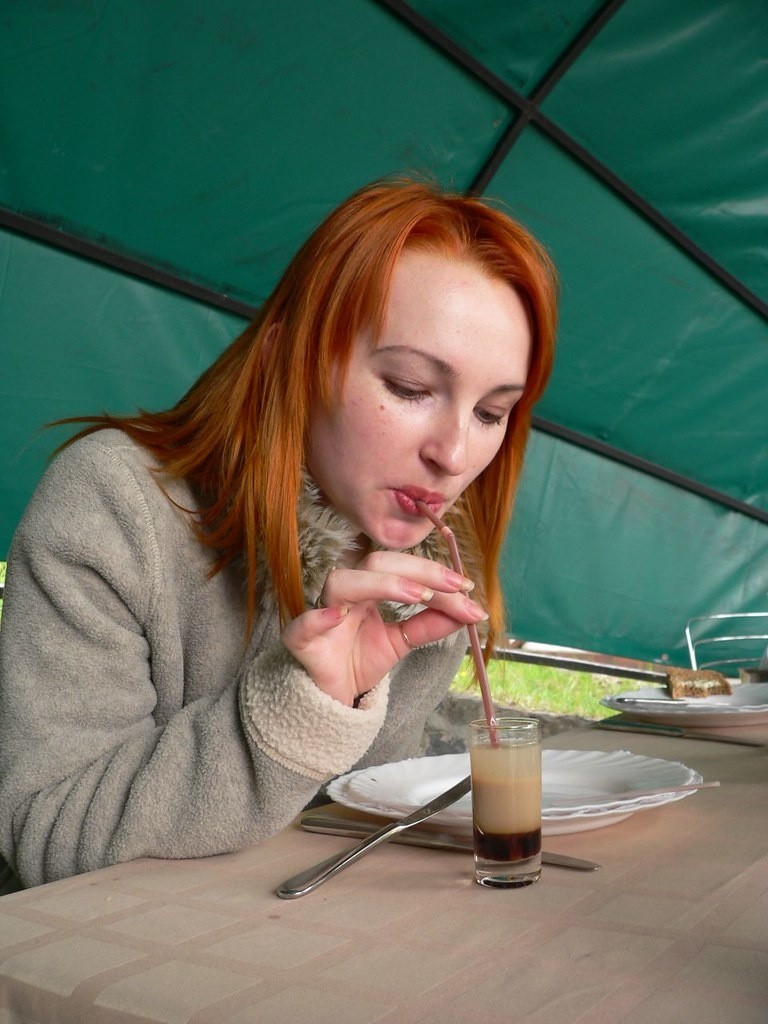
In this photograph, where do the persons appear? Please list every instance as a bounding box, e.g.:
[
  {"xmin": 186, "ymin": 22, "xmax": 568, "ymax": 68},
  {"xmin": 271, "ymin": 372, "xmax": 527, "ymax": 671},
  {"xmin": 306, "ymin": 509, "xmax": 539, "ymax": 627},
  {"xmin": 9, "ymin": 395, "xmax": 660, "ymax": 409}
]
[{"xmin": 0, "ymin": 181, "xmax": 559, "ymax": 893}]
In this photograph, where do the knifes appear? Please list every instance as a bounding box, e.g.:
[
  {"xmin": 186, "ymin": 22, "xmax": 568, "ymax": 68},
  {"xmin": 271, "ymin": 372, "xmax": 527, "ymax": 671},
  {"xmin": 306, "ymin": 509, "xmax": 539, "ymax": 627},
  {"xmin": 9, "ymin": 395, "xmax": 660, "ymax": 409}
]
[
  {"xmin": 597, "ymin": 718, "xmax": 766, "ymax": 751},
  {"xmin": 302, "ymin": 814, "xmax": 603, "ymax": 874},
  {"xmin": 274, "ymin": 777, "xmax": 473, "ymax": 899}
]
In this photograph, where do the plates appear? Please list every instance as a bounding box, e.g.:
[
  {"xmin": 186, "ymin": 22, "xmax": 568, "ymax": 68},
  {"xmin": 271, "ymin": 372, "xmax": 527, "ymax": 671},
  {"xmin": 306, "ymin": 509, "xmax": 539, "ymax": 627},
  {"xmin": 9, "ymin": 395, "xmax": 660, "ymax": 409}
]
[
  {"xmin": 325, "ymin": 748, "xmax": 702, "ymax": 835},
  {"xmin": 597, "ymin": 678, "xmax": 768, "ymax": 729}
]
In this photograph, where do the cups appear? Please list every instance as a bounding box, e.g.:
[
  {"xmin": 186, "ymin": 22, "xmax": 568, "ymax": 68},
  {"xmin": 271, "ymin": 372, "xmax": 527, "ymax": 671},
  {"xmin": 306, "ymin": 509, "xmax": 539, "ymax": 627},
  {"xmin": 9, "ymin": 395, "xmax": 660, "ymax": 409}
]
[{"xmin": 468, "ymin": 716, "xmax": 544, "ymax": 889}]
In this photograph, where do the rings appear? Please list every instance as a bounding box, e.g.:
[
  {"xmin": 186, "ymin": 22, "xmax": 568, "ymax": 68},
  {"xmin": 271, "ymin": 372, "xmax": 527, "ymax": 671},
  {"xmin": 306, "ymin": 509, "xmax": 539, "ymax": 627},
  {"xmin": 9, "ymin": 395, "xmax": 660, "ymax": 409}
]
[{"xmin": 399, "ymin": 620, "xmax": 417, "ymax": 650}]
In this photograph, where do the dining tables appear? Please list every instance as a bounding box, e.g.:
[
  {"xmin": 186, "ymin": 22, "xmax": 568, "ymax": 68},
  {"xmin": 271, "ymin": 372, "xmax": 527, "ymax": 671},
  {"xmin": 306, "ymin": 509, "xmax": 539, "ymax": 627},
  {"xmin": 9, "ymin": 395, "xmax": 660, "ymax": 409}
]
[{"xmin": 0, "ymin": 724, "xmax": 768, "ymax": 1024}]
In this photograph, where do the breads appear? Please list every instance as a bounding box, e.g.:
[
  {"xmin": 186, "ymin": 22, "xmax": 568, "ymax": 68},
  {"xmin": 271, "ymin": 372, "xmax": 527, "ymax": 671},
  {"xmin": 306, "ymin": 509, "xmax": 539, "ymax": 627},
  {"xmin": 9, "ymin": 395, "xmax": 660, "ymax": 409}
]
[{"xmin": 665, "ymin": 667, "xmax": 732, "ymax": 700}]
